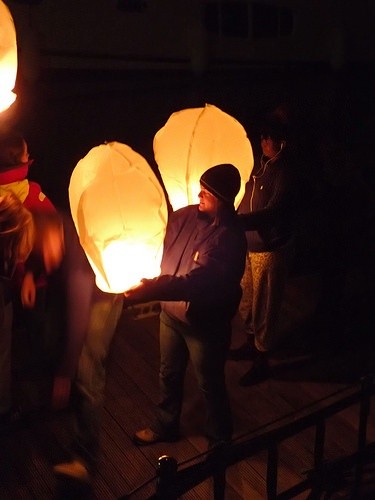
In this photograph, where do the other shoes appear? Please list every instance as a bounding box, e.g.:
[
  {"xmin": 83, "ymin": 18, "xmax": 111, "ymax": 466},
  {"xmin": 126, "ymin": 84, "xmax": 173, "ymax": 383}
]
[
  {"xmin": 54, "ymin": 462, "xmax": 88, "ymax": 483},
  {"xmin": 239, "ymin": 362, "xmax": 272, "ymax": 387},
  {"xmin": 132, "ymin": 428, "xmax": 157, "ymax": 445},
  {"xmin": 228, "ymin": 345, "xmax": 255, "ymax": 360}
]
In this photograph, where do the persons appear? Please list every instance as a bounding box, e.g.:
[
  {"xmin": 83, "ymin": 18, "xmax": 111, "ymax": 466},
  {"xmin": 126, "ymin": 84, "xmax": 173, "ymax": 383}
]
[
  {"xmin": 230, "ymin": 122, "xmax": 308, "ymax": 370},
  {"xmin": 119, "ymin": 164, "xmax": 250, "ymax": 449},
  {"xmin": 0, "ymin": 137, "xmax": 69, "ymax": 408}
]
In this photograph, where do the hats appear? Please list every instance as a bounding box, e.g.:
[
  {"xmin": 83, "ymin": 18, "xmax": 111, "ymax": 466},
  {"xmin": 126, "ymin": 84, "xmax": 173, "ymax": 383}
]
[{"xmin": 200, "ymin": 164, "xmax": 241, "ymax": 203}]
